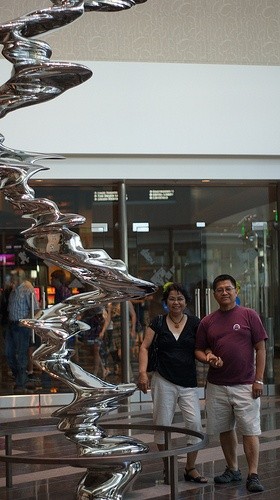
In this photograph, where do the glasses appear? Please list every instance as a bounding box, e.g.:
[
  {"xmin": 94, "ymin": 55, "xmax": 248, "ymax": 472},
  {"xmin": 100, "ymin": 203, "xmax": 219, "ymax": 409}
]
[{"xmin": 214, "ymin": 287, "xmax": 235, "ymax": 293}]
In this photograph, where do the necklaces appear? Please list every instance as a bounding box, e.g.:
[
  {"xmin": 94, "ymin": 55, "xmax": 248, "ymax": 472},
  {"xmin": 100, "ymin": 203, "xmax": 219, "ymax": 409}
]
[{"xmin": 169, "ymin": 313, "xmax": 184, "ymax": 328}]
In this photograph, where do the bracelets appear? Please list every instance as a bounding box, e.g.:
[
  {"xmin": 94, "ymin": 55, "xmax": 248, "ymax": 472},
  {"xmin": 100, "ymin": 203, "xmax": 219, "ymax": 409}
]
[{"xmin": 256, "ymin": 380, "xmax": 264, "ymax": 385}]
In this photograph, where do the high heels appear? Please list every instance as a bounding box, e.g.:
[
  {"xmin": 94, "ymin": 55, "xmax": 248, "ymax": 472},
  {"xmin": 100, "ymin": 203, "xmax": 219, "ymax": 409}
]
[
  {"xmin": 184, "ymin": 468, "xmax": 207, "ymax": 483},
  {"xmin": 163, "ymin": 469, "xmax": 170, "ymax": 485}
]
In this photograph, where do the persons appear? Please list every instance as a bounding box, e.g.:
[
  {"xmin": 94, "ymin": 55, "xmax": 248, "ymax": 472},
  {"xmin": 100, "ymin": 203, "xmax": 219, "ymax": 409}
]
[
  {"xmin": 5, "ymin": 269, "xmax": 39, "ymax": 391},
  {"xmin": 50, "ymin": 269, "xmax": 153, "ymax": 382},
  {"xmin": 194, "ymin": 274, "xmax": 268, "ymax": 492},
  {"xmin": 137, "ymin": 283, "xmax": 223, "ymax": 484}
]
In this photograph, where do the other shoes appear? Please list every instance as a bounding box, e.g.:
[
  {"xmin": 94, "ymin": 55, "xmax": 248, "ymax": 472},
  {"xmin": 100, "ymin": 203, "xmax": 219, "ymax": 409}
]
[
  {"xmin": 8, "ymin": 369, "xmax": 13, "ymax": 376},
  {"xmin": 13, "ymin": 385, "xmax": 25, "ymax": 393}
]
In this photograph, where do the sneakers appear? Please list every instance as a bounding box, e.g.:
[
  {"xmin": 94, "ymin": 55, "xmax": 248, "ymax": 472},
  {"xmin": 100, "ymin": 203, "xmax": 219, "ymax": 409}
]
[
  {"xmin": 246, "ymin": 473, "xmax": 264, "ymax": 492},
  {"xmin": 214, "ymin": 468, "xmax": 242, "ymax": 484}
]
